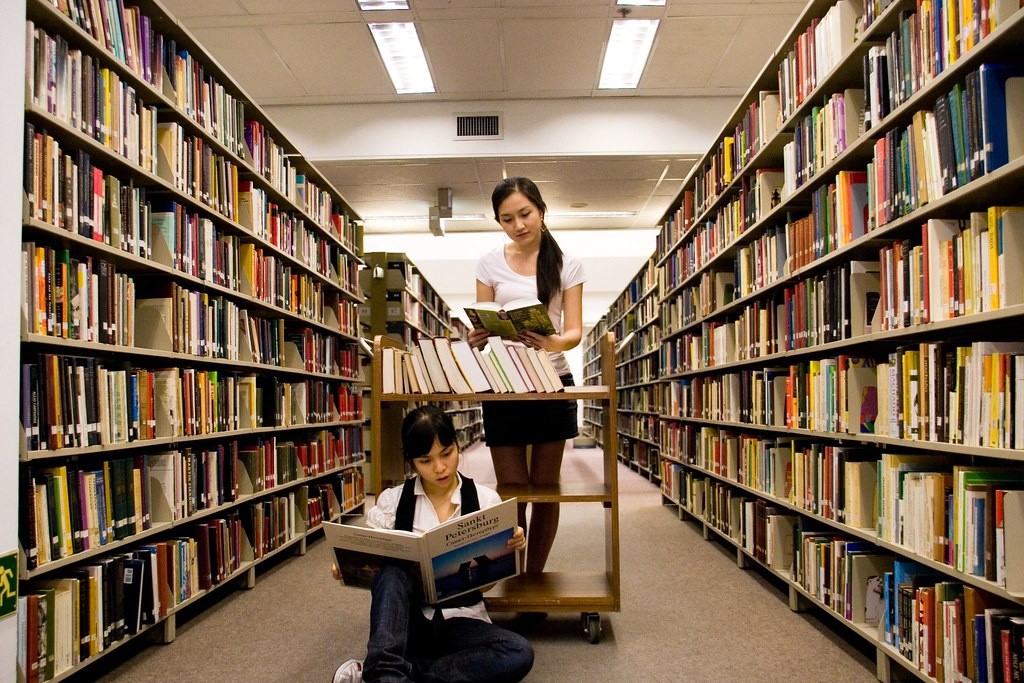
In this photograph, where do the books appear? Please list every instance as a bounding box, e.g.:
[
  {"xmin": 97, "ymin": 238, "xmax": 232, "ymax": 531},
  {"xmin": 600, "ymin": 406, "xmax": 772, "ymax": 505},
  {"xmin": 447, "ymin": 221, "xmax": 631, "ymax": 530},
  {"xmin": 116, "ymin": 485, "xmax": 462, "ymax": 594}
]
[
  {"xmin": 419, "ymin": 402, "xmax": 483, "ymax": 448},
  {"xmin": 583, "ymin": 0, "xmax": 1024, "ymax": 680},
  {"xmin": 380, "ymin": 261, "xmax": 565, "ymax": 396},
  {"xmin": 322, "ymin": 498, "xmax": 520, "ymax": 602},
  {"xmin": 15, "ymin": 0, "xmax": 365, "ymax": 683},
  {"xmin": 463, "ymin": 298, "xmax": 556, "ymax": 342}
]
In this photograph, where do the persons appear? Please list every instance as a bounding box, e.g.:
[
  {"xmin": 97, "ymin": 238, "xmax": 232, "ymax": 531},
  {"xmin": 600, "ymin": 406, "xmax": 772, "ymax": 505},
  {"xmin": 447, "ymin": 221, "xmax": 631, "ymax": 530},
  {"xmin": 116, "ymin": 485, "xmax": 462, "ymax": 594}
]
[
  {"xmin": 331, "ymin": 407, "xmax": 534, "ymax": 683},
  {"xmin": 469, "ymin": 178, "xmax": 588, "ymax": 620}
]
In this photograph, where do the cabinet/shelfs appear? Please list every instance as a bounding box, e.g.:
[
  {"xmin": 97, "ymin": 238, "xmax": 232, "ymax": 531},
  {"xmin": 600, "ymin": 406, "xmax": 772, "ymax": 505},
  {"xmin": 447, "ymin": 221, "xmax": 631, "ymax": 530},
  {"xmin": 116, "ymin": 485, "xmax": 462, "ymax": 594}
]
[
  {"xmin": 583, "ymin": 251, "xmax": 663, "ymax": 484},
  {"xmin": 656, "ymin": 1, "xmax": 1024, "ymax": 683},
  {"xmin": 360, "ymin": 252, "xmax": 485, "ymax": 506},
  {"xmin": 19, "ymin": 0, "xmax": 368, "ymax": 682},
  {"xmin": 360, "ymin": 331, "xmax": 621, "ymax": 612}
]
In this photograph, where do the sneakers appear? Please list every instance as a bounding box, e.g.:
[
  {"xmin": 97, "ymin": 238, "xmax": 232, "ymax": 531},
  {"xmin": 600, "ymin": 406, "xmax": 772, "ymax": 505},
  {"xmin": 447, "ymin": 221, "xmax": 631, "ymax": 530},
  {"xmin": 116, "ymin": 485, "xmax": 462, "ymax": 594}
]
[{"xmin": 331, "ymin": 659, "xmax": 365, "ymax": 683}]
